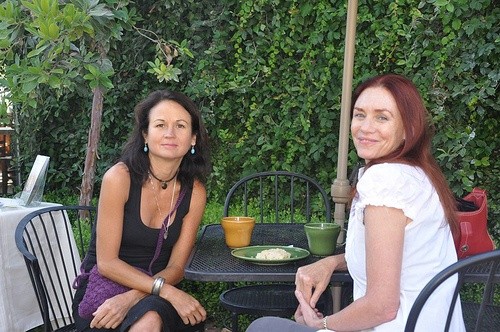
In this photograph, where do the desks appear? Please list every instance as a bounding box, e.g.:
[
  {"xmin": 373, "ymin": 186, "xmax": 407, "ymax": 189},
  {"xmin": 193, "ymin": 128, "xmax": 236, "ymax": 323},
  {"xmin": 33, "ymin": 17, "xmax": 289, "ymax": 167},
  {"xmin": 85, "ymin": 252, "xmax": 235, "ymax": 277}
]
[{"xmin": 0, "ymin": 197, "xmax": 82, "ymax": 332}]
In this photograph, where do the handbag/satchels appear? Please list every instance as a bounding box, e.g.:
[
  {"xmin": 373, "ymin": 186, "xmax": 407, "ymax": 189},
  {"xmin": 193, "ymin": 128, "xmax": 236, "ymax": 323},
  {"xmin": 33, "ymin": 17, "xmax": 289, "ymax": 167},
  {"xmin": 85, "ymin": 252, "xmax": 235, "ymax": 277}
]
[
  {"xmin": 450, "ymin": 186, "xmax": 496, "ymax": 259},
  {"xmin": 78, "ymin": 262, "xmax": 155, "ymax": 319}
]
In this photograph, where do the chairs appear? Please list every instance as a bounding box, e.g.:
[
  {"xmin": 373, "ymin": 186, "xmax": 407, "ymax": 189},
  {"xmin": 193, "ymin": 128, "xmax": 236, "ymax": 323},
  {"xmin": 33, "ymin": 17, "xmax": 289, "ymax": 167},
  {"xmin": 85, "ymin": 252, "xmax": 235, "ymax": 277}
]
[
  {"xmin": 404, "ymin": 247, "xmax": 500, "ymax": 332},
  {"xmin": 15, "ymin": 206, "xmax": 96, "ymax": 332},
  {"xmin": 220, "ymin": 171, "xmax": 329, "ymax": 332}
]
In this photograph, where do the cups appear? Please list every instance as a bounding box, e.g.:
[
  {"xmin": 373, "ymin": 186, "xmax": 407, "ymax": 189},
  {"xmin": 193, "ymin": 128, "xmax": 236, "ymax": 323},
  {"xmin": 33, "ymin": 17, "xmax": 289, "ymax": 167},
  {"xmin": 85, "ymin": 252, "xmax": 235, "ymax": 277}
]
[
  {"xmin": 304, "ymin": 223, "xmax": 347, "ymax": 257},
  {"xmin": 220, "ymin": 217, "xmax": 256, "ymax": 250}
]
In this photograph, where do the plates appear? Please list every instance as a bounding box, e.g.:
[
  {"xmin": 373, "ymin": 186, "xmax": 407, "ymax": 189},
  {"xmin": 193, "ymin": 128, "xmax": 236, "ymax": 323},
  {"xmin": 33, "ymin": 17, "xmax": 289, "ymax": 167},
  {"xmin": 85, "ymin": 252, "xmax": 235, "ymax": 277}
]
[{"xmin": 230, "ymin": 246, "xmax": 310, "ymax": 265}]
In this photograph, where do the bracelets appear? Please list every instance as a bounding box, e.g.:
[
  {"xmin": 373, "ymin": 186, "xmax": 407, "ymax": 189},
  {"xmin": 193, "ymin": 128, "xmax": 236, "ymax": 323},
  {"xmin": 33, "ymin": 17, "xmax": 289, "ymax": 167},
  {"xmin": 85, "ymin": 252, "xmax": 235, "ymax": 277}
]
[
  {"xmin": 323, "ymin": 316, "xmax": 328, "ymax": 330},
  {"xmin": 151, "ymin": 276, "xmax": 166, "ymax": 296}
]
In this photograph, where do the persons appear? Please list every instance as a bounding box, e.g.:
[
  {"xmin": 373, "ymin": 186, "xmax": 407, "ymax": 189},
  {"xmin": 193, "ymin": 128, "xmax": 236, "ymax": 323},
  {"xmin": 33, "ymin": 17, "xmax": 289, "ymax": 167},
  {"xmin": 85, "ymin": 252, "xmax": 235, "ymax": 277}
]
[
  {"xmin": 244, "ymin": 73, "xmax": 466, "ymax": 332},
  {"xmin": 71, "ymin": 88, "xmax": 207, "ymax": 332}
]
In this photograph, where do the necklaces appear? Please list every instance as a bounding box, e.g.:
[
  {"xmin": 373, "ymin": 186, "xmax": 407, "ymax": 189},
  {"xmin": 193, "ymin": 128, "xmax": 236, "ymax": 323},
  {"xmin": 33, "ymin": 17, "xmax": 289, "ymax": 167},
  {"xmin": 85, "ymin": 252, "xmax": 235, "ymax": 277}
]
[
  {"xmin": 149, "ymin": 168, "xmax": 178, "ymax": 190},
  {"xmin": 150, "ymin": 178, "xmax": 177, "ymax": 240}
]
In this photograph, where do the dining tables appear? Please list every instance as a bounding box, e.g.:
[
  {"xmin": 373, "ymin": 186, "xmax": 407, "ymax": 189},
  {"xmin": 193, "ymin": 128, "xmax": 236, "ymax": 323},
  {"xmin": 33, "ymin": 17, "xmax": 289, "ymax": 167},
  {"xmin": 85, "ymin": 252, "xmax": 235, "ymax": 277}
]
[{"xmin": 184, "ymin": 223, "xmax": 354, "ymax": 332}]
